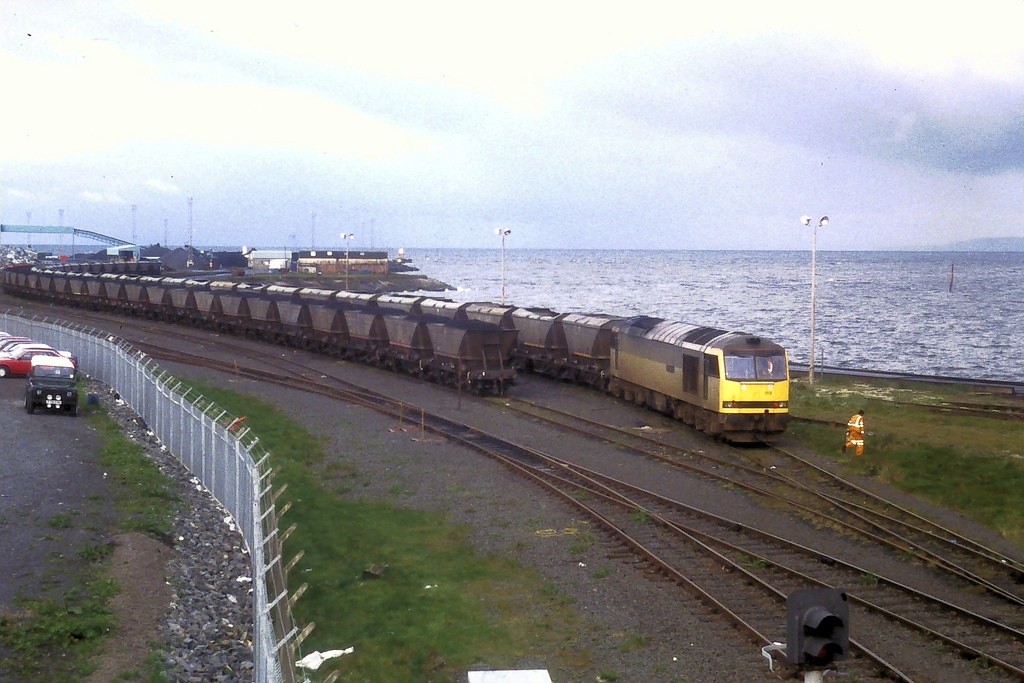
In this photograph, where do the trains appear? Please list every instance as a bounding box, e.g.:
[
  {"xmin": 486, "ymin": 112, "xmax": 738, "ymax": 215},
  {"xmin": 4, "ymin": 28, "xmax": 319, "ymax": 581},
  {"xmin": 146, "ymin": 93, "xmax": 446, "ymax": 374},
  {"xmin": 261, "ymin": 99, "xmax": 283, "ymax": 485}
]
[{"xmin": 0, "ymin": 259, "xmax": 791, "ymax": 449}]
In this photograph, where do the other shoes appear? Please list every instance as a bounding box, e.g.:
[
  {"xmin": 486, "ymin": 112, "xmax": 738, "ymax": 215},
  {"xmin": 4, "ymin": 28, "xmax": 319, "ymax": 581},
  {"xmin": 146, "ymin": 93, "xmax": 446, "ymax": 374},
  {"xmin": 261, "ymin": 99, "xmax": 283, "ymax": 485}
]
[{"xmin": 842, "ymin": 445, "xmax": 846, "ymax": 453}]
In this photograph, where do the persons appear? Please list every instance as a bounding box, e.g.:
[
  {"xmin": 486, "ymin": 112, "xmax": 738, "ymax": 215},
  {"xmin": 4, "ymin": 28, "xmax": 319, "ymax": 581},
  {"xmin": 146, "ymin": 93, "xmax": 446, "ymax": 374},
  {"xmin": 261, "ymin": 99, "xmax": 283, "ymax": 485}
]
[
  {"xmin": 765, "ymin": 357, "xmax": 772, "ymax": 374},
  {"xmin": 842, "ymin": 410, "xmax": 865, "ymax": 456}
]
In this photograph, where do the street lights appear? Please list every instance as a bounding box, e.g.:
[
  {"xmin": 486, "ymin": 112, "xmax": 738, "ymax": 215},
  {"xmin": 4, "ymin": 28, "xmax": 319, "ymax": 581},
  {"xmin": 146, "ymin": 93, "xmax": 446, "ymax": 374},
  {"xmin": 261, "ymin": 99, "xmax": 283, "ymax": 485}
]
[
  {"xmin": 494, "ymin": 226, "xmax": 512, "ymax": 304},
  {"xmin": 340, "ymin": 233, "xmax": 355, "ymax": 291},
  {"xmin": 800, "ymin": 214, "xmax": 830, "ymax": 382}
]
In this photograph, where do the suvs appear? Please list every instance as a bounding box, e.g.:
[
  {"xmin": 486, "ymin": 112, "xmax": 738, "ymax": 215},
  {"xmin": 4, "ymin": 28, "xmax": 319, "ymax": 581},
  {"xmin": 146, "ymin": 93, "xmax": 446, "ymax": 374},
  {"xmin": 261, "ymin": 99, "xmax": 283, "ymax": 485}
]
[{"xmin": 22, "ymin": 354, "xmax": 77, "ymax": 416}]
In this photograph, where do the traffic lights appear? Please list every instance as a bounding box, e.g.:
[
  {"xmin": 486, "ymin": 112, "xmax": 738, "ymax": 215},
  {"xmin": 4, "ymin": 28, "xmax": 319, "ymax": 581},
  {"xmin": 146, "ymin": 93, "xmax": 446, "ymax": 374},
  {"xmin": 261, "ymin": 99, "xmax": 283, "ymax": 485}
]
[{"xmin": 786, "ymin": 585, "xmax": 851, "ymax": 669}]
